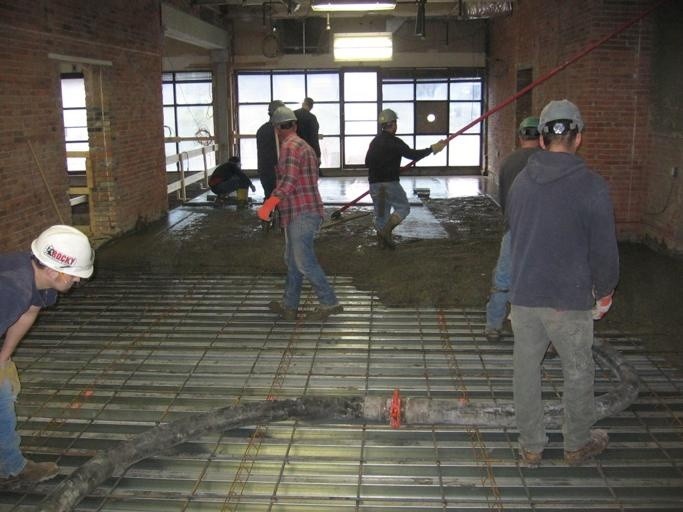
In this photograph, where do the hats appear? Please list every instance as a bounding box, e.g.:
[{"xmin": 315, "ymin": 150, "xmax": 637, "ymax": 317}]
[
  {"xmin": 538, "ymin": 100, "xmax": 584, "ymax": 135},
  {"xmin": 517, "ymin": 115, "xmax": 538, "ymax": 133}
]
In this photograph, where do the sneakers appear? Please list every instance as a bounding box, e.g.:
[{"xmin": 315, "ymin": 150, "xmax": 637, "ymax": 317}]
[
  {"xmin": 304, "ymin": 305, "xmax": 343, "ymax": 320},
  {"xmin": 485, "ymin": 329, "xmax": 499, "ymax": 337},
  {"xmin": 565, "ymin": 428, "xmax": 610, "ymax": 466},
  {"xmin": 518, "ymin": 443, "xmax": 543, "ymax": 465},
  {"xmin": 0, "ymin": 460, "xmax": 60, "ymax": 494},
  {"xmin": 544, "ymin": 342, "xmax": 557, "ymax": 360},
  {"xmin": 268, "ymin": 301, "xmax": 297, "ymax": 321}
]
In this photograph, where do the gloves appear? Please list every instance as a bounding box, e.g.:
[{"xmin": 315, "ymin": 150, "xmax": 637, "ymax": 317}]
[
  {"xmin": 592, "ymin": 284, "xmax": 615, "ymax": 321},
  {"xmin": 431, "ymin": 139, "xmax": 448, "ymax": 155},
  {"xmin": 258, "ymin": 196, "xmax": 281, "ymax": 222},
  {"xmin": 0, "ymin": 356, "xmax": 21, "ymax": 396}
]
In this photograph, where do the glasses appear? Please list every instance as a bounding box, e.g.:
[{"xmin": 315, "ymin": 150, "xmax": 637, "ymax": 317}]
[{"xmin": 60, "ymin": 248, "xmax": 95, "ymax": 270}]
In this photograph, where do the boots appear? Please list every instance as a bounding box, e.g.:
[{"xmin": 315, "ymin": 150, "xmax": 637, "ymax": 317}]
[{"xmin": 374, "ymin": 214, "xmax": 401, "ymax": 248}]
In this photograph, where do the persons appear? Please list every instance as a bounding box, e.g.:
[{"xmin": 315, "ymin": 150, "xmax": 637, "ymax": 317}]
[
  {"xmin": 257, "ymin": 105, "xmax": 344, "ymax": 322},
  {"xmin": 506, "ymin": 99, "xmax": 622, "ymax": 469},
  {"xmin": 0, "ymin": 224, "xmax": 95, "ymax": 492},
  {"xmin": 293, "ymin": 99, "xmax": 324, "ymax": 171},
  {"xmin": 208, "ymin": 155, "xmax": 257, "ymax": 206},
  {"xmin": 366, "ymin": 109, "xmax": 447, "ymax": 248},
  {"xmin": 483, "ymin": 116, "xmax": 555, "ymax": 355},
  {"xmin": 257, "ymin": 99, "xmax": 288, "ymax": 225}
]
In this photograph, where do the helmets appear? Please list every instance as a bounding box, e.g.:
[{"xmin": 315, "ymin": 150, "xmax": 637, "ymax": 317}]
[
  {"xmin": 31, "ymin": 224, "xmax": 95, "ymax": 279},
  {"xmin": 269, "ymin": 100, "xmax": 298, "ymax": 125},
  {"xmin": 379, "ymin": 109, "xmax": 397, "ymax": 126}
]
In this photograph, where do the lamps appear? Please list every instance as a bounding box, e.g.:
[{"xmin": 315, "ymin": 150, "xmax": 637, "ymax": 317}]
[{"xmin": 311, "ymin": 0, "xmax": 397, "ymax": 12}]
